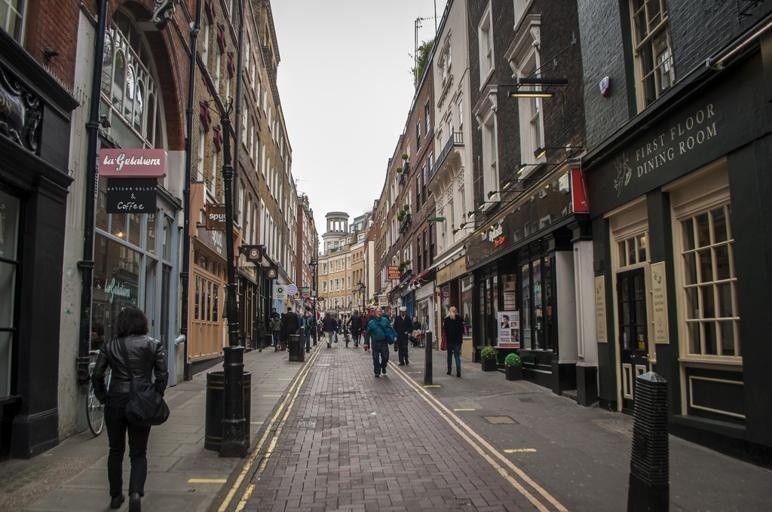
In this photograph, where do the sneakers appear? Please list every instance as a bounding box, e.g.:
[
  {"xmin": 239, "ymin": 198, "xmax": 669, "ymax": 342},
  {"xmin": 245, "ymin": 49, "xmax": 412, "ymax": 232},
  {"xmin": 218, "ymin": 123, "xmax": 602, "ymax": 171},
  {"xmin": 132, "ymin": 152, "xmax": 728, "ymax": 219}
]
[
  {"xmin": 398, "ymin": 359, "xmax": 410, "ymax": 366},
  {"xmin": 110, "ymin": 491, "xmax": 126, "ymax": 509},
  {"xmin": 375, "ymin": 371, "xmax": 380, "ymax": 377},
  {"xmin": 446, "ymin": 368, "xmax": 461, "ymax": 378},
  {"xmin": 381, "ymin": 365, "xmax": 386, "ymax": 374},
  {"xmin": 129, "ymin": 491, "xmax": 142, "ymax": 512}
]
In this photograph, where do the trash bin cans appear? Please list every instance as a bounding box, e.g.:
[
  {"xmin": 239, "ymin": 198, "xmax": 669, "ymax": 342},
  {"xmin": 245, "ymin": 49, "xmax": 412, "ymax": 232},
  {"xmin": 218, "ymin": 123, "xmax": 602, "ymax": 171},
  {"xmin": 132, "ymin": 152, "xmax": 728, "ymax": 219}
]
[
  {"xmin": 204, "ymin": 370, "xmax": 251, "ymax": 450},
  {"xmin": 289, "ymin": 334, "xmax": 306, "ymax": 361}
]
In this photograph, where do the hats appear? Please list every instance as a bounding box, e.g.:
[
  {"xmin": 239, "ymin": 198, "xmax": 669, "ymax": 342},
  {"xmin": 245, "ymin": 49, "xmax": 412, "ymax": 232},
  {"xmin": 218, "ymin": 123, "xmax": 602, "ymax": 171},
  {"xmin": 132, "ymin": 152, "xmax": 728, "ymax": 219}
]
[{"xmin": 400, "ymin": 306, "xmax": 408, "ymax": 312}]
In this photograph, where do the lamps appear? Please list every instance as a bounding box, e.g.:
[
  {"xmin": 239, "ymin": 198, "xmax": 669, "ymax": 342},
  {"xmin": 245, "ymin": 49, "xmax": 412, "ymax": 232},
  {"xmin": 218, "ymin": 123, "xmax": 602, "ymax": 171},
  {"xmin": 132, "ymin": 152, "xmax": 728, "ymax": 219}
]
[
  {"xmin": 452, "ymin": 144, "xmax": 589, "ymax": 236},
  {"xmin": 491, "ymin": 30, "xmax": 578, "ymax": 113}
]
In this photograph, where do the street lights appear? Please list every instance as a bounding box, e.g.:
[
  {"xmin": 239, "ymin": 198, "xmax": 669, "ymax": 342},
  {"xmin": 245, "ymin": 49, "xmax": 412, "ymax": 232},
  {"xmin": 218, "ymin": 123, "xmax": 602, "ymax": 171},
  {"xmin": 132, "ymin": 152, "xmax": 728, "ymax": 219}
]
[
  {"xmin": 309, "ymin": 256, "xmax": 317, "ymax": 345},
  {"xmin": 357, "ymin": 282, "xmax": 366, "ymax": 317}
]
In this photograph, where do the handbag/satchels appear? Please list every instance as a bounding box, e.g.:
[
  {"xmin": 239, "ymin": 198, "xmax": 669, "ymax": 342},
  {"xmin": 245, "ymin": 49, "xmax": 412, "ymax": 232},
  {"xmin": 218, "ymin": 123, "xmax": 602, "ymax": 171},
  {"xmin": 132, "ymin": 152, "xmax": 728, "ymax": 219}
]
[
  {"xmin": 385, "ymin": 328, "xmax": 398, "ymax": 344},
  {"xmin": 439, "ymin": 335, "xmax": 448, "ymax": 350},
  {"xmin": 124, "ymin": 384, "xmax": 170, "ymax": 426}
]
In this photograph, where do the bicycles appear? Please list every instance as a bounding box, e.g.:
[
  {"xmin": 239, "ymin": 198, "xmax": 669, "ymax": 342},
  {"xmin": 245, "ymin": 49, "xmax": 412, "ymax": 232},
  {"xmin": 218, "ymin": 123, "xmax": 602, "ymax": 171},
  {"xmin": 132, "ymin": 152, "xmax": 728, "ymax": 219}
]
[{"xmin": 85, "ymin": 347, "xmax": 112, "ymax": 440}]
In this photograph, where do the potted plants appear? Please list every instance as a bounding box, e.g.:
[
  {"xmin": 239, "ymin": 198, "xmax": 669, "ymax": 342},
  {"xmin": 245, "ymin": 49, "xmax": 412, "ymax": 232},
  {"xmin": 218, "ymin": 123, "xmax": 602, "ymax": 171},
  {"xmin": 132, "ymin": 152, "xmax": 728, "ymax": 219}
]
[
  {"xmin": 480, "ymin": 344, "xmax": 497, "ymax": 371},
  {"xmin": 505, "ymin": 352, "xmax": 525, "ymax": 381}
]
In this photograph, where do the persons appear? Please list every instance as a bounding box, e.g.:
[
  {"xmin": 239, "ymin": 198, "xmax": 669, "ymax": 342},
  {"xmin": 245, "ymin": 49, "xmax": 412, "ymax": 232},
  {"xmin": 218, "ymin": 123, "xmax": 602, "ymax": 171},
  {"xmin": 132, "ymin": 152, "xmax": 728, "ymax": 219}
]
[
  {"xmin": 444, "ymin": 306, "xmax": 464, "ymax": 377},
  {"xmin": 465, "ymin": 313, "xmax": 471, "ymax": 336},
  {"xmin": 269, "ymin": 305, "xmax": 421, "ymax": 377},
  {"xmin": 501, "ymin": 315, "xmax": 509, "ymax": 329},
  {"xmin": 91, "ymin": 306, "xmax": 168, "ymax": 512}
]
